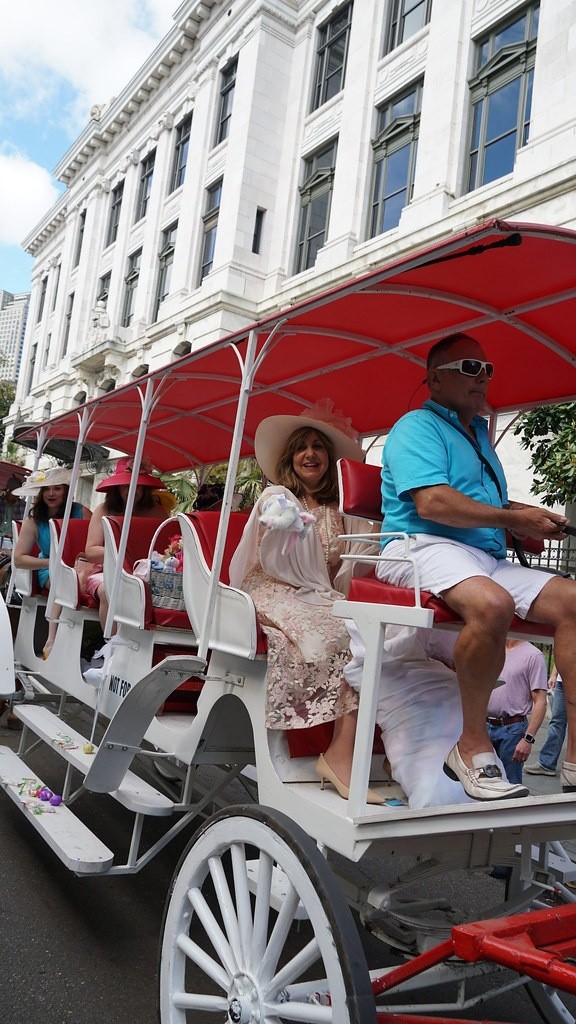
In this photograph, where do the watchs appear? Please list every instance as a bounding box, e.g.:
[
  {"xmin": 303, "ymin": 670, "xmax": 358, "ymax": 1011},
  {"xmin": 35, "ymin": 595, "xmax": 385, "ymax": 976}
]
[{"xmin": 522, "ymin": 733, "xmax": 535, "ymax": 744}]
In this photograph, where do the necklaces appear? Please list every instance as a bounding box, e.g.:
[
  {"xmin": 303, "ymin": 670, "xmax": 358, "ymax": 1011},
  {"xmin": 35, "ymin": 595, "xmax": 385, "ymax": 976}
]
[{"xmin": 301, "ymin": 495, "xmax": 329, "ymax": 566}]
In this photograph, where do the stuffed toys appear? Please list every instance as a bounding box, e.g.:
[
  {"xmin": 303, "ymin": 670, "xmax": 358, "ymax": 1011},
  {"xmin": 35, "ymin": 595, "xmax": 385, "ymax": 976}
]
[
  {"xmin": 259, "ymin": 496, "xmax": 303, "ymax": 532},
  {"xmin": 163, "ymin": 556, "xmax": 179, "ymax": 572}
]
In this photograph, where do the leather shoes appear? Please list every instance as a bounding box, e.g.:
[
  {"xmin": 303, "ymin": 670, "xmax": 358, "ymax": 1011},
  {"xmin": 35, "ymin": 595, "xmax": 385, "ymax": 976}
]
[
  {"xmin": 560, "ymin": 761, "xmax": 576, "ymax": 793},
  {"xmin": 443, "ymin": 741, "xmax": 529, "ymax": 801}
]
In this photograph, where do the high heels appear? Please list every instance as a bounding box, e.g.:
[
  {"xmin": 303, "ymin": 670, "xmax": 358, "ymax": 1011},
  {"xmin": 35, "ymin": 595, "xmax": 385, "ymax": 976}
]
[
  {"xmin": 315, "ymin": 753, "xmax": 386, "ymax": 803},
  {"xmin": 382, "ymin": 757, "xmax": 392, "ymax": 786}
]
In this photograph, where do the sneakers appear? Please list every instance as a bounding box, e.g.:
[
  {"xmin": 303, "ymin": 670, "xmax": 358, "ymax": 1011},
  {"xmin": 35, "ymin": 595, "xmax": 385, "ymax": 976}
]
[{"xmin": 524, "ymin": 763, "xmax": 557, "ymax": 776}]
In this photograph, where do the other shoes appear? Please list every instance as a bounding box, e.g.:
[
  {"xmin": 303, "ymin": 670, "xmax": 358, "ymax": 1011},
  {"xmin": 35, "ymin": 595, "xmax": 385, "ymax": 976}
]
[{"xmin": 42, "ymin": 647, "xmax": 48, "ymax": 660}]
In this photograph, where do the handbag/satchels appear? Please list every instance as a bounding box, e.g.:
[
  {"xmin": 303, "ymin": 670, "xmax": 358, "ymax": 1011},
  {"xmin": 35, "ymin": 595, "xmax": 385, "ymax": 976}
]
[{"xmin": 131, "ymin": 559, "xmax": 151, "ymax": 582}]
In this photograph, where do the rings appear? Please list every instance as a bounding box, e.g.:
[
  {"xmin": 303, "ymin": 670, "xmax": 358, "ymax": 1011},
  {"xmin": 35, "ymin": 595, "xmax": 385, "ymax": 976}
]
[{"xmin": 522, "ymin": 758, "xmax": 525, "ymax": 760}]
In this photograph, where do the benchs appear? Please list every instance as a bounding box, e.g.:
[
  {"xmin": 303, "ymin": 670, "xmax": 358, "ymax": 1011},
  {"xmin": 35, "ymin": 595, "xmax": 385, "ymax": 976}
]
[{"xmin": 3, "ymin": 458, "xmax": 576, "ymax": 863}]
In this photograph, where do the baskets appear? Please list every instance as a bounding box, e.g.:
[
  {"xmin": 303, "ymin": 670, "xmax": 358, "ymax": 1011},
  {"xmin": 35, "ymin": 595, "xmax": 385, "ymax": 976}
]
[{"xmin": 147, "ymin": 517, "xmax": 186, "ymax": 611}]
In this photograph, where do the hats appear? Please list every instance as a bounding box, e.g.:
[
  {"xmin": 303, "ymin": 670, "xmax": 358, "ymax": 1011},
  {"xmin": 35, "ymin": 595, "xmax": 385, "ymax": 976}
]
[
  {"xmin": 193, "ymin": 482, "xmax": 243, "ymax": 511},
  {"xmin": 10, "ymin": 467, "xmax": 82, "ymax": 496},
  {"xmin": 95, "ymin": 454, "xmax": 167, "ymax": 492},
  {"xmin": 0, "ymin": 478, "xmax": 23, "ymax": 492},
  {"xmin": 254, "ymin": 397, "xmax": 368, "ymax": 485}
]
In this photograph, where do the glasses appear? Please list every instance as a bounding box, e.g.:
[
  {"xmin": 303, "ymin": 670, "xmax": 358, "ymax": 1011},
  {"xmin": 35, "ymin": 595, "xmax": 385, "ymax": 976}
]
[{"xmin": 435, "ymin": 358, "xmax": 494, "ymax": 379}]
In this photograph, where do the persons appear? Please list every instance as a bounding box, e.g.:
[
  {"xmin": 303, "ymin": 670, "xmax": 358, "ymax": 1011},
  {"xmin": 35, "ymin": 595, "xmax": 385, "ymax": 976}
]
[
  {"xmin": 193, "ymin": 485, "xmax": 241, "ymax": 513},
  {"xmin": 376, "ymin": 332, "xmax": 576, "ymax": 801},
  {"xmin": 523, "ymin": 663, "xmax": 568, "ymax": 776},
  {"xmin": 0, "ymin": 474, "xmax": 27, "ymax": 592},
  {"xmin": 225, "ymin": 413, "xmax": 393, "ymax": 805},
  {"xmin": 12, "ymin": 466, "xmax": 93, "ymax": 660},
  {"xmin": 484, "ymin": 637, "xmax": 547, "ymax": 785},
  {"xmin": 77, "ymin": 456, "xmax": 167, "ymax": 644}
]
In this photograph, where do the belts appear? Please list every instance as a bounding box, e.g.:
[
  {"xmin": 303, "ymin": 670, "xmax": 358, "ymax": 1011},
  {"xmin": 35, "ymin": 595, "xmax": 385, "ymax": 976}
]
[{"xmin": 486, "ymin": 715, "xmax": 526, "ymax": 726}]
